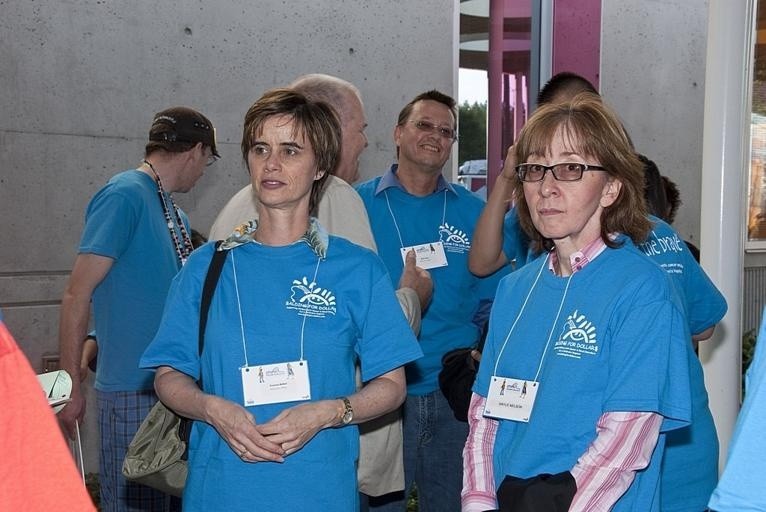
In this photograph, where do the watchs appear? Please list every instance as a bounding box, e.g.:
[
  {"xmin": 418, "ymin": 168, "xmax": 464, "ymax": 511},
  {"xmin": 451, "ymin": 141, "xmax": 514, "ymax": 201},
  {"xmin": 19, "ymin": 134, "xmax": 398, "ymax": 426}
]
[{"xmin": 334, "ymin": 396, "xmax": 353, "ymax": 429}]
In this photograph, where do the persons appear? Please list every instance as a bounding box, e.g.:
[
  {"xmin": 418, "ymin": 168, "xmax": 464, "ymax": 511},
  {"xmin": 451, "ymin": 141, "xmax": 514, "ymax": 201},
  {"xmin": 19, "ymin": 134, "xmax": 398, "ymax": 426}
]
[
  {"xmin": 205, "ymin": 74, "xmax": 434, "ymax": 498},
  {"xmin": 462, "ymin": 73, "xmax": 729, "ymax": 512},
  {"xmin": 135, "ymin": 89, "xmax": 424, "ymax": 512},
  {"xmin": 351, "ymin": 89, "xmax": 514, "ymax": 512},
  {"xmin": 703, "ymin": 309, "xmax": 765, "ymax": 512},
  {"xmin": 56, "ymin": 106, "xmax": 221, "ymax": 512}
]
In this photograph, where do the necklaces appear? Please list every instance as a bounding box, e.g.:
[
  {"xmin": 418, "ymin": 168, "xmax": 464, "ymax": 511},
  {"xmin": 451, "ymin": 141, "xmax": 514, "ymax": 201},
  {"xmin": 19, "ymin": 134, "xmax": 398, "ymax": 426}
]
[{"xmin": 142, "ymin": 158, "xmax": 194, "ymax": 265}]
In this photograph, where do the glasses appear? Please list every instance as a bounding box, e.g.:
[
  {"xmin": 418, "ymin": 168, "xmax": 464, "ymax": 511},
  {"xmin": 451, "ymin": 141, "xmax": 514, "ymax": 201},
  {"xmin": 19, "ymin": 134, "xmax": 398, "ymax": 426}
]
[
  {"xmin": 406, "ymin": 120, "xmax": 459, "ymax": 141},
  {"xmin": 201, "ymin": 145, "xmax": 217, "ymax": 168},
  {"xmin": 513, "ymin": 161, "xmax": 613, "ymax": 183}
]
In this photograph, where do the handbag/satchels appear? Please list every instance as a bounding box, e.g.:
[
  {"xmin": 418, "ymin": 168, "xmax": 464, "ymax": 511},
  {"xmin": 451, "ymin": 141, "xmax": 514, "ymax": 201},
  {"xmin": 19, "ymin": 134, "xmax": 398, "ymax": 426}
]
[{"xmin": 120, "ymin": 398, "xmax": 192, "ymax": 498}]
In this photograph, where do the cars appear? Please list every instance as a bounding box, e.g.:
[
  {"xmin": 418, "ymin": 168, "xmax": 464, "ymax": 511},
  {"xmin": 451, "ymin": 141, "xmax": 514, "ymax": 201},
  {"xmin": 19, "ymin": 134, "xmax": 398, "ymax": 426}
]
[{"xmin": 458, "ymin": 159, "xmax": 489, "ymax": 203}]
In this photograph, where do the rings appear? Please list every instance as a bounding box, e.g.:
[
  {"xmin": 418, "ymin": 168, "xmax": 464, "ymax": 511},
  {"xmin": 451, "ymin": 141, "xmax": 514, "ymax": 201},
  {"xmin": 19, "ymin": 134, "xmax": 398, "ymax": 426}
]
[{"xmin": 241, "ymin": 448, "xmax": 247, "ymax": 456}]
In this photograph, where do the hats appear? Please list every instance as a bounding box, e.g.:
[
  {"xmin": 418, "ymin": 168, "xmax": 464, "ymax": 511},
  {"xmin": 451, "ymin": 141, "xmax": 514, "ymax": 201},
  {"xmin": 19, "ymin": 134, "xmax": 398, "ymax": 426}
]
[{"xmin": 148, "ymin": 106, "xmax": 222, "ymax": 159}]
